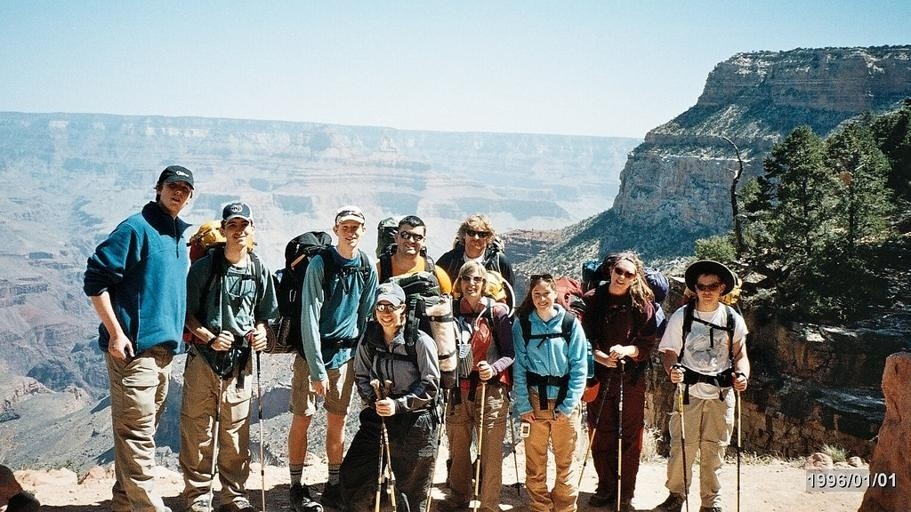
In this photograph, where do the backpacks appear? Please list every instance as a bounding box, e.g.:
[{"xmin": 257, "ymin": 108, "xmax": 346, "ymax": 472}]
[
  {"xmin": 270, "ymin": 229, "xmax": 334, "ymax": 319},
  {"xmin": 382, "ymin": 273, "xmax": 474, "ymax": 390}
]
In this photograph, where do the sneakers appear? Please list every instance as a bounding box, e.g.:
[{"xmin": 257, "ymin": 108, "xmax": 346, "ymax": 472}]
[
  {"xmin": 649, "ymin": 490, "xmax": 686, "ymax": 511},
  {"xmin": 587, "ymin": 482, "xmax": 618, "ymax": 508},
  {"xmin": 319, "ymin": 481, "xmax": 349, "ymax": 512},
  {"xmin": 434, "ymin": 496, "xmax": 471, "ymax": 511},
  {"xmin": 184, "ymin": 499, "xmax": 216, "ymax": 512},
  {"xmin": 286, "ymin": 483, "xmax": 327, "ymax": 511},
  {"xmin": 220, "ymin": 496, "xmax": 257, "ymax": 512}
]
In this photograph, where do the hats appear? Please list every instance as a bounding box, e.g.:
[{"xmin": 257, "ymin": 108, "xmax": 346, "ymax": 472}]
[
  {"xmin": 684, "ymin": 260, "xmax": 737, "ymax": 297},
  {"xmin": 221, "ymin": 199, "xmax": 255, "ymax": 225},
  {"xmin": 334, "ymin": 204, "xmax": 367, "ymax": 226},
  {"xmin": 157, "ymin": 164, "xmax": 196, "ymax": 191},
  {"xmin": 374, "ymin": 282, "xmax": 409, "ymax": 307}
]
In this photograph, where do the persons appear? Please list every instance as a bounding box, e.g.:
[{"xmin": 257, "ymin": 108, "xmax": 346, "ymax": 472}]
[
  {"xmin": 653, "ymin": 259, "xmax": 750, "ymax": 512},
  {"xmin": 580, "ymin": 250, "xmax": 657, "ymax": 512},
  {"xmin": 375, "ymin": 215, "xmax": 452, "ymax": 299},
  {"xmin": 179, "ymin": 202, "xmax": 279, "ymax": 512},
  {"xmin": 286, "ymin": 204, "xmax": 379, "ymax": 511},
  {"xmin": 81, "ymin": 164, "xmax": 194, "ymax": 512},
  {"xmin": 334, "ymin": 281, "xmax": 445, "ymax": 512},
  {"xmin": 436, "ymin": 258, "xmax": 515, "ymax": 512},
  {"xmin": 434, "ymin": 210, "xmax": 516, "ymax": 494},
  {"xmin": 510, "ymin": 272, "xmax": 589, "ymax": 512}
]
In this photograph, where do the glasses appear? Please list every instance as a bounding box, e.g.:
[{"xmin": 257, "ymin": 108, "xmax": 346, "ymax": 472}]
[
  {"xmin": 695, "ymin": 282, "xmax": 721, "ymax": 290},
  {"xmin": 529, "ymin": 274, "xmax": 554, "ymax": 280},
  {"xmin": 399, "ymin": 230, "xmax": 425, "ymax": 241},
  {"xmin": 376, "ymin": 303, "xmax": 402, "ymax": 313},
  {"xmin": 613, "ymin": 267, "xmax": 637, "ymax": 281},
  {"xmin": 460, "ymin": 274, "xmax": 483, "ymax": 283},
  {"xmin": 464, "ymin": 229, "xmax": 489, "ymax": 238}
]
